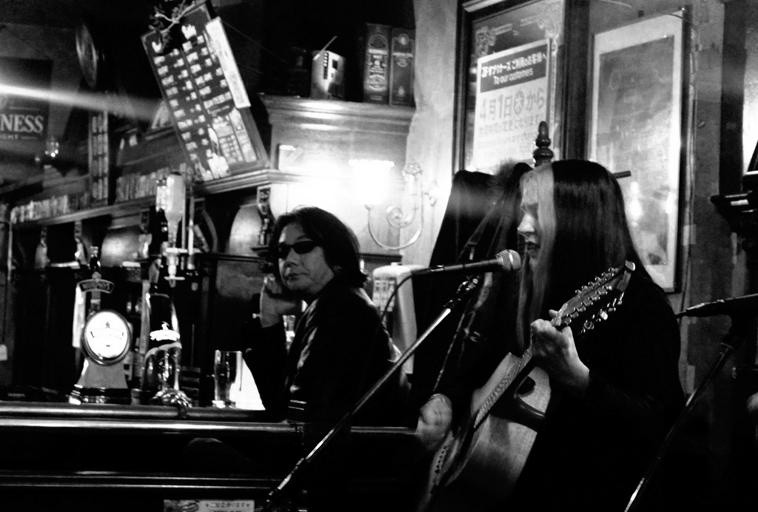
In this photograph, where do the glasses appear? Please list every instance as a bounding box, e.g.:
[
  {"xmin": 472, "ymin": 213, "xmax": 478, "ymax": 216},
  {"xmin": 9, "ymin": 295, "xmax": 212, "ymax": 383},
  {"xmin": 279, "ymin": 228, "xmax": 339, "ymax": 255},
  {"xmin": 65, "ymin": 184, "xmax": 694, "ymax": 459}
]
[{"xmin": 277, "ymin": 241, "xmax": 316, "ymax": 259}]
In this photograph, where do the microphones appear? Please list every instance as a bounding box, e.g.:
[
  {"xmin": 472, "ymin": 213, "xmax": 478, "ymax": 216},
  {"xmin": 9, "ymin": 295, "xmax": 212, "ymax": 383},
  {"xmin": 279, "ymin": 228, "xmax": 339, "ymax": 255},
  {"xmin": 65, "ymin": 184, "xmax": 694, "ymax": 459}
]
[
  {"xmin": 686, "ymin": 294, "xmax": 758, "ymax": 318},
  {"xmin": 413, "ymin": 249, "xmax": 522, "ymax": 279}
]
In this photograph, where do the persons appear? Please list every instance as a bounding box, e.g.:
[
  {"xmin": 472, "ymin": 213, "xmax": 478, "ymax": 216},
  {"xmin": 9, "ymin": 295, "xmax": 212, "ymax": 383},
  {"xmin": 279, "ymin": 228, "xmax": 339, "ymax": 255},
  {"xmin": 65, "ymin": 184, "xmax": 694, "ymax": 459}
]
[
  {"xmin": 246, "ymin": 206, "xmax": 407, "ymax": 426},
  {"xmin": 415, "ymin": 157, "xmax": 686, "ymax": 512}
]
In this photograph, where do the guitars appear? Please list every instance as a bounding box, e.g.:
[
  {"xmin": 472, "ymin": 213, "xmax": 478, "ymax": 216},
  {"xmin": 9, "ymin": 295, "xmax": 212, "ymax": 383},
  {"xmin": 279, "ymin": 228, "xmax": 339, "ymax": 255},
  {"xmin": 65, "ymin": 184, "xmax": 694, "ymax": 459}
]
[{"xmin": 419, "ymin": 260, "xmax": 635, "ymax": 512}]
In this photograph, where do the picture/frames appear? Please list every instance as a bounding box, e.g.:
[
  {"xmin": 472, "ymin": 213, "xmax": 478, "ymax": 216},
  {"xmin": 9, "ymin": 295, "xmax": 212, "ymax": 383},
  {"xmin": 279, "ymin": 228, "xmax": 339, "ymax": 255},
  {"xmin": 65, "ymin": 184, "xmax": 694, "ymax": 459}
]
[
  {"xmin": 584, "ymin": 6, "xmax": 689, "ymax": 293},
  {"xmin": 452, "ymin": 1, "xmax": 589, "ymax": 179}
]
